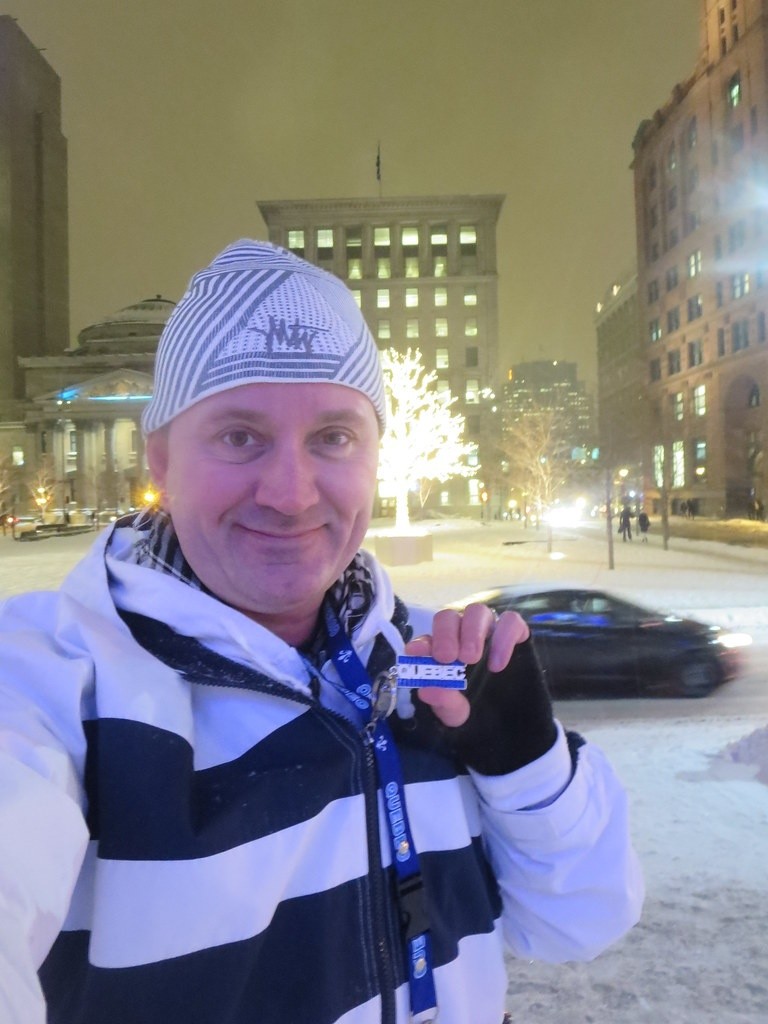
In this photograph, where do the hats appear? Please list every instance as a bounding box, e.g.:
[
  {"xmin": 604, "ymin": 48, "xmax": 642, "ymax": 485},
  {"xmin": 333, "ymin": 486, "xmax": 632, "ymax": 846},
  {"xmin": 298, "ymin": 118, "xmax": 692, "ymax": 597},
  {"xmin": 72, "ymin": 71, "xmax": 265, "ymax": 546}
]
[{"xmin": 140, "ymin": 237, "xmax": 387, "ymax": 440}]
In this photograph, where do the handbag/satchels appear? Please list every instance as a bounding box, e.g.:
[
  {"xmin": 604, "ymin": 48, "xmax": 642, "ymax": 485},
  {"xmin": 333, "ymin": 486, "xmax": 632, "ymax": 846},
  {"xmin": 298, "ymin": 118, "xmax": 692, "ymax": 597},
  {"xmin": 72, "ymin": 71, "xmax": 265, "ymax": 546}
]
[{"xmin": 618, "ymin": 523, "xmax": 623, "ymax": 534}]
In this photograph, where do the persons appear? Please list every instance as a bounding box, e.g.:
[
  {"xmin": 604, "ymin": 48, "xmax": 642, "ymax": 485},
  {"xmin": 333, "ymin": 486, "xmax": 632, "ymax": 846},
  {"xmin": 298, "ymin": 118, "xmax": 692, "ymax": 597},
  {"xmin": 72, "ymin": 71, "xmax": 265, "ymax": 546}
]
[
  {"xmin": 617, "ymin": 505, "xmax": 650, "ymax": 543},
  {"xmin": 0, "ymin": 238, "xmax": 647, "ymax": 1024},
  {"xmin": 746, "ymin": 496, "xmax": 764, "ymax": 521},
  {"xmin": 679, "ymin": 498, "xmax": 696, "ymax": 518}
]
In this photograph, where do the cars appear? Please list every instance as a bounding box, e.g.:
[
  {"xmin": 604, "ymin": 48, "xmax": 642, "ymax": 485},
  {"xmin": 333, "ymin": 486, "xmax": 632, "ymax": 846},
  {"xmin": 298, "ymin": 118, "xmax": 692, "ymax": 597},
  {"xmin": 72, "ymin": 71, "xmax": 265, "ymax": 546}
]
[{"xmin": 465, "ymin": 586, "xmax": 735, "ymax": 700}]
